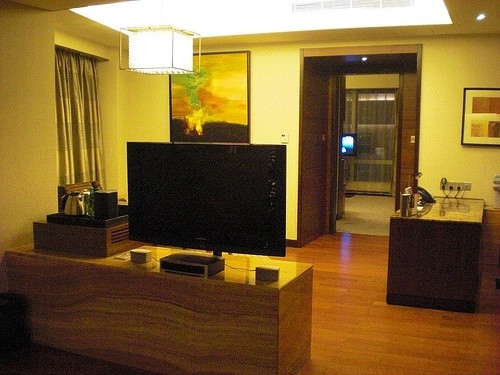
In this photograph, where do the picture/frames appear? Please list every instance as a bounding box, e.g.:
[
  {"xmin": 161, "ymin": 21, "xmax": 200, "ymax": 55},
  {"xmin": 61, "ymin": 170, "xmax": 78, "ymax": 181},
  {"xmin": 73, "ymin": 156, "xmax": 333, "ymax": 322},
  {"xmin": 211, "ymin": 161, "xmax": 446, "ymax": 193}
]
[
  {"xmin": 169, "ymin": 50, "xmax": 251, "ymax": 143},
  {"xmin": 461, "ymin": 87, "xmax": 500, "ymax": 149}
]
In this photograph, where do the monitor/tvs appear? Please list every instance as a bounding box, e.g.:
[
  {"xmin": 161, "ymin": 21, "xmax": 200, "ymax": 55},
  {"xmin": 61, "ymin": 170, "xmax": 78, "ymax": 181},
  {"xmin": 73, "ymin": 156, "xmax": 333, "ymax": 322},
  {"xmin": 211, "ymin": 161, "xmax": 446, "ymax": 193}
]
[
  {"xmin": 126, "ymin": 140, "xmax": 287, "ymax": 259},
  {"xmin": 341, "ymin": 133, "xmax": 358, "ymax": 156}
]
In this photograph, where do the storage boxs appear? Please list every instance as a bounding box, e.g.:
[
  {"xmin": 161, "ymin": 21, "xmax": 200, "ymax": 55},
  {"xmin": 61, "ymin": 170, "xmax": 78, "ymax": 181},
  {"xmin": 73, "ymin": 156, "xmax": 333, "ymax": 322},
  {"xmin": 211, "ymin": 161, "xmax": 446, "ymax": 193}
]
[
  {"xmin": 158, "ymin": 253, "xmax": 226, "ymax": 278},
  {"xmin": 31, "ymin": 222, "xmax": 146, "ymax": 259},
  {"xmin": 94, "ymin": 190, "xmax": 119, "ymax": 221},
  {"xmin": 47, "ymin": 205, "xmax": 129, "ymax": 227}
]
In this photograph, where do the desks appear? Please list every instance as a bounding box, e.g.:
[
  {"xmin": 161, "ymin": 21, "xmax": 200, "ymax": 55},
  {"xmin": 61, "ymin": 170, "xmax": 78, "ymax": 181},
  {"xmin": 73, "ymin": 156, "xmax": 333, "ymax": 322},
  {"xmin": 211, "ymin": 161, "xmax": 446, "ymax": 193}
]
[
  {"xmin": 3, "ymin": 242, "xmax": 314, "ymax": 375},
  {"xmin": 386, "ymin": 197, "xmax": 486, "ymax": 314}
]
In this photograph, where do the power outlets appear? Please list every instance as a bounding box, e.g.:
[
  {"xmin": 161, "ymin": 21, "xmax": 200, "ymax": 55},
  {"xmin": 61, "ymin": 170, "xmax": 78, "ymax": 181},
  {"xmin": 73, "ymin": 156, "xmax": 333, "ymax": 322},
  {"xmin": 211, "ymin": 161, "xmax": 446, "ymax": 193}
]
[
  {"xmin": 448, "ymin": 182, "xmax": 456, "ymax": 191},
  {"xmin": 463, "ymin": 183, "xmax": 472, "ymax": 190},
  {"xmin": 455, "ymin": 182, "xmax": 464, "ymax": 191},
  {"xmin": 440, "ymin": 181, "xmax": 448, "ymax": 190}
]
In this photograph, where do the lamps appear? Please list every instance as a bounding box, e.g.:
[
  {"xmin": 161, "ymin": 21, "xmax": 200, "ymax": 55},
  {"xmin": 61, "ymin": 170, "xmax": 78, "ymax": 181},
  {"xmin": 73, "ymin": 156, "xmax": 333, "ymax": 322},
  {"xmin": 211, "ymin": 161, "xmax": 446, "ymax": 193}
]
[{"xmin": 119, "ymin": 25, "xmax": 202, "ymax": 75}]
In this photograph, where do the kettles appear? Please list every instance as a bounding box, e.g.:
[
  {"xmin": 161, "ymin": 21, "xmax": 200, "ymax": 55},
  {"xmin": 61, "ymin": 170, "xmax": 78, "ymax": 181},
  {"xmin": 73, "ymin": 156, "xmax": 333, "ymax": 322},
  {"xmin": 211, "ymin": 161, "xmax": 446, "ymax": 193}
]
[{"xmin": 61, "ymin": 192, "xmax": 86, "ymax": 216}]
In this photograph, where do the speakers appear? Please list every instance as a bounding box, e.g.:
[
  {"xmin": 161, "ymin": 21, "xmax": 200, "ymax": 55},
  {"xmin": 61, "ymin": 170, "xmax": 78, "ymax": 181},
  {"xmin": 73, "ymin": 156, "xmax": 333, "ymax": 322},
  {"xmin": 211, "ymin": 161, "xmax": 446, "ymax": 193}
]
[{"xmin": 95, "ymin": 190, "xmax": 119, "ymax": 220}]
